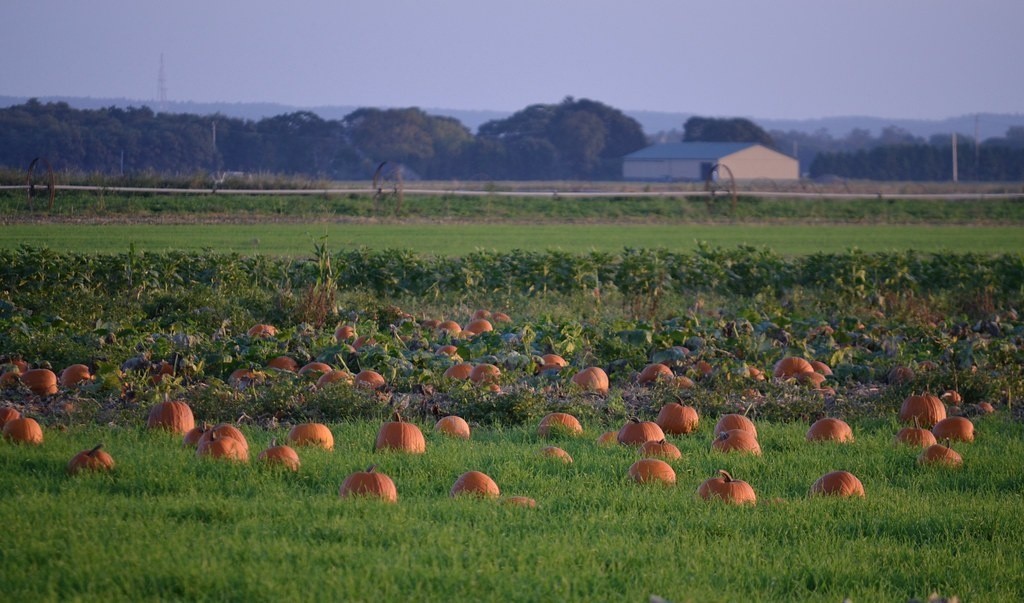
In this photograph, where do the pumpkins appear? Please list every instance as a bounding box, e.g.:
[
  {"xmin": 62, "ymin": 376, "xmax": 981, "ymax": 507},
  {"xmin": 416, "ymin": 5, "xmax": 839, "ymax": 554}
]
[
  {"xmin": 0, "ymin": 309, "xmax": 538, "ymax": 509},
  {"xmin": 534, "ymin": 310, "xmax": 1018, "ymax": 505}
]
